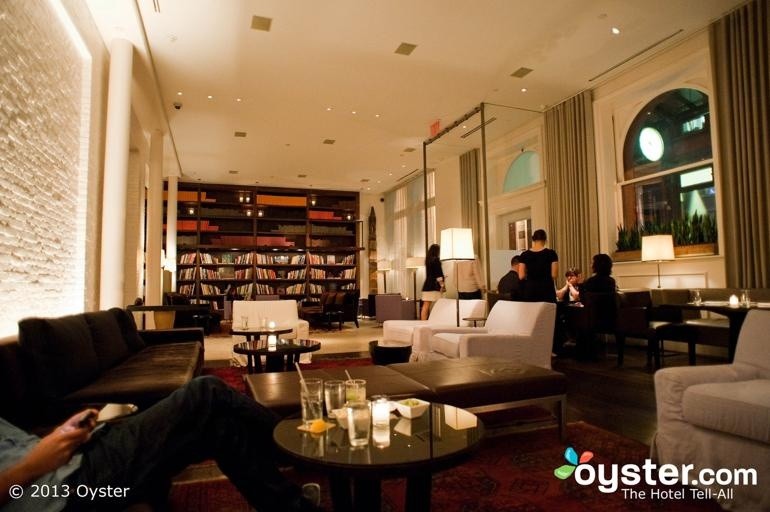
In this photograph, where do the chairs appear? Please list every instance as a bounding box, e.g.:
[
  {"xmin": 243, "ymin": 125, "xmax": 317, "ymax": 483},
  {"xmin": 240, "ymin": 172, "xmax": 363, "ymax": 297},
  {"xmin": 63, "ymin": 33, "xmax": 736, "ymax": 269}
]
[{"xmin": 487, "ymin": 288, "xmax": 510, "ymax": 314}]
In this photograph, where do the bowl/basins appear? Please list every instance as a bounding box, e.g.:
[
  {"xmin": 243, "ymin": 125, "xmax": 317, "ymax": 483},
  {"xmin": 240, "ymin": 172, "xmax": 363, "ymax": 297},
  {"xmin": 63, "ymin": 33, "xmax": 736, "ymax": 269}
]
[
  {"xmin": 396, "ymin": 399, "xmax": 430, "ymax": 419},
  {"xmin": 394, "ymin": 419, "xmax": 427, "ymax": 436}
]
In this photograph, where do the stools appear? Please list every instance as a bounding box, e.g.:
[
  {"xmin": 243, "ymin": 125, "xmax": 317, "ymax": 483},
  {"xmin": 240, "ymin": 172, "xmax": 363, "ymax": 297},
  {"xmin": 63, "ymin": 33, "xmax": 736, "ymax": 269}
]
[{"xmin": 369, "ymin": 340, "xmax": 413, "ymax": 366}]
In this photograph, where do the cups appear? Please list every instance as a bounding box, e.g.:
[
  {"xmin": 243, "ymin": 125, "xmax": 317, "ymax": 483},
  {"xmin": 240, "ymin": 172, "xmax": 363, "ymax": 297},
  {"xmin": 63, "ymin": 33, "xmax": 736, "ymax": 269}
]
[
  {"xmin": 347, "ymin": 403, "xmax": 370, "ymax": 448},
  {"xmin": 345, "ymin": 380, "xmax": 367, "ymax": 402},
  {"xmin": 302, "ymin": 433, "xmax": 325, "ymax": 458},
  {"xmin": 741, "ymin": 289, "xmax": 751, "ymax": 307},
  {"xmin": 373, "ymin": 427, "xmax": 391, "ymax": 450},
  {"xmin": 691, "ymin": 289, "xmax": 702, "ymax": 307},
  {"xmin": 240, "ymin": 314, "xmax": 249, "ymax": 331},
  {"xmin": 372, "ymin": 400, "xmax": 390, "ymax": 428},
  {"xmin": 348, "ymin": 446, "xmax": 371, "ymax": 464},
  {"xmin": 300, "ymin": 379, "xmax": 323, "ymax": 425},
  {"xmin": 325, "ymin": 381, "xmax": 345, "ymax": 418}
]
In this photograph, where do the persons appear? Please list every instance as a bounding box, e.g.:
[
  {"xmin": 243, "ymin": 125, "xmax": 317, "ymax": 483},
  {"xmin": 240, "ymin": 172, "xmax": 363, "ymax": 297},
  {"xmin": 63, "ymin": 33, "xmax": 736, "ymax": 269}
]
[
  {"xmin": 453, "ymin": 255, "xmax": 487, "ymax": 300},
  {"xmin": 498, "ymin": 255, "xmax": 522, "ymax": 302},
  {"xmin": 0, "ymin": 375, "xmax": 325, "ymax": 512},
  {"xmin": 558, "ymin": 270, "xmax": 581, "ymax": 303},
  {"xmin": 420, "ymin": 243, "xmax": 446, "ymax": 321},
  {"xmin": 519, "ymin": 229, "xmax": 559, "ymax": 359},
  {"xmin": 579, "ymin": 254, "xmax": 617, "ymax": 364}
]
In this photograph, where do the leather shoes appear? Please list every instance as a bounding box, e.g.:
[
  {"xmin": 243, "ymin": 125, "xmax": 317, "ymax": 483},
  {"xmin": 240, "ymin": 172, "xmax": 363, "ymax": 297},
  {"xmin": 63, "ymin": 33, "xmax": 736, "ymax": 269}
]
[{"xmin": 552, "ymin": 353, "xmax": 557, "ymax": 356}]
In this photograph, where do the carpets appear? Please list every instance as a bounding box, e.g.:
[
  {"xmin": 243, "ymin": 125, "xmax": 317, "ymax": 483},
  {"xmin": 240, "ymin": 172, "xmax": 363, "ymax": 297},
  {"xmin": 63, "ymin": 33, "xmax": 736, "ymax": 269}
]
[{"xmin": 172, "ymin": 350, "xmax": 730, "ymax": 512}]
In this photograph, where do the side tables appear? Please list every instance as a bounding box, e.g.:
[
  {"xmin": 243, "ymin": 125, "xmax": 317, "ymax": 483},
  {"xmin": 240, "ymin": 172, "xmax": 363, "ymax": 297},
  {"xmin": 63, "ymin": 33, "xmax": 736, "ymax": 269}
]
[{"xmin": 462, "ymin": 318, "xmax": 487, "ymax": 327}]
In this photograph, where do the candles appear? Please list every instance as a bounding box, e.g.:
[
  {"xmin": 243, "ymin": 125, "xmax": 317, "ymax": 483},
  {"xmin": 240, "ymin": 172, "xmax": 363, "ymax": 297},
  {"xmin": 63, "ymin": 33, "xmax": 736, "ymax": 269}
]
[
  {"xmin": 729, "ymin": 294, "xmax": 738, "ymax": 306},
  {"xmin": 372, "ymin": 398, "xmax": 392, "ymax": 426},
  {"xmin": 268, "ymin": 319, "xmax": 276, "ymax": 329},
  {"xmin": 372, "ymin": 426, "xmax": 390, "ymax": 449},
  {"xmin": 267, "ymin": 333, "xmax": 277, "ymax": 347}
]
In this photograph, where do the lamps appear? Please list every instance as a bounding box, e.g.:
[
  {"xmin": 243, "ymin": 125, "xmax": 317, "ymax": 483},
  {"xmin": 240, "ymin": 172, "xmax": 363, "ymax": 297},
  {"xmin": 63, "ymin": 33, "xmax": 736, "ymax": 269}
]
[
  {"xmin": 440, "ymin": 228, "xmax": 476, "ymax": 327},
  {"xmin": 164, "ymin": 256, "xmax": 176, "ymax": 273},
  {"xmin": 377, "ymin": 261, "xmax": 392, "ymax": 294},
  {"xmin": 404, "ymin": 257, "xmax": 425, "ymax": 320},
  {"xmin": 640, "ymin": 234, "xmax": 676, "ymax": 288},
  {"xmin": 161, "ymin": 248, "xmax": 166, "ymax": 269}
]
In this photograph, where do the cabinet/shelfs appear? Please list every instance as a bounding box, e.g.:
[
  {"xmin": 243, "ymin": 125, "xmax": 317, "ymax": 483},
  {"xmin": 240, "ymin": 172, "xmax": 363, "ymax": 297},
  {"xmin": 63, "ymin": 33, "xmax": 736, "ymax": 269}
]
[{"xmin": 143, "ymin": 181, "xmax": 361, "ymax": 320}]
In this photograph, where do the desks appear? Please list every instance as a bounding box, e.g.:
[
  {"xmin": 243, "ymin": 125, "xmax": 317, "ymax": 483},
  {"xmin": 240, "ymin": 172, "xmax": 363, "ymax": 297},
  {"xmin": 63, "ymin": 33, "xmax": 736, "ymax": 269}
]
[{"xmin": 127, "ymin": 305, "xmax": 176, "ymax": 330}]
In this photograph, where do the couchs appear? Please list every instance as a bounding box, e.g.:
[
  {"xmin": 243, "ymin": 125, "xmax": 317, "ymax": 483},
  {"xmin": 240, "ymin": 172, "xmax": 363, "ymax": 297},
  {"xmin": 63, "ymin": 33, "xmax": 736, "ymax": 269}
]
[
  {"xmin": 17, "ymin": 307, "xmax": 205, "ymax": 411},
  {"xmin": 383, "ymin": 297, "xmax": 488, "ymax": 345},
  {"xmin": 240, "ymin": 358, "xmax": 567, "ymax": 440},
  {"xmin": 301, "ymin": 290, "xmax": 361, "ymax": 331},
  {"xmin": 649, "ymin": 288, "xmax": 770, "ymax": 323},
  {"xmin": 165, "ymin": 291, "xmax": 223, "ymax": 331},
  {"xmin": 409, "ymin": 300, "xmax": 557, "ymax": 370},
  {"xmin": 375, "ymin": 294, "xmax": 415, "ymax": 327},
  {"xmin": 653, "ymin": 309, "xmax": 770, "ymax": 512},
  {"xmin": 232, "ymin": 299, "xmax": 309, "ymax": 344},
  {"xmin": 617, "ymin": 291, "xmax": 683, "ymax": 367}
]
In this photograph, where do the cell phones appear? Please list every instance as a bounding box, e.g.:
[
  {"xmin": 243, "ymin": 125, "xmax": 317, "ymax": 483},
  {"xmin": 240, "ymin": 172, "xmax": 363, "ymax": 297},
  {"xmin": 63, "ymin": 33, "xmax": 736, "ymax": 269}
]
[{"xmin": 79, "ymin": 411, "xmax": 97, "ymax": 428}]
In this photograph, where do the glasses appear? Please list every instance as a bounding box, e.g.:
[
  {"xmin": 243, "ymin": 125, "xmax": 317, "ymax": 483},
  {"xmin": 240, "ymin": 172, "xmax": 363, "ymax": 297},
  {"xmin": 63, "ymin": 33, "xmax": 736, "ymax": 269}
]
[{"xmin": 566, "ymin": 273, "xmax": 574, "ymax": 276}]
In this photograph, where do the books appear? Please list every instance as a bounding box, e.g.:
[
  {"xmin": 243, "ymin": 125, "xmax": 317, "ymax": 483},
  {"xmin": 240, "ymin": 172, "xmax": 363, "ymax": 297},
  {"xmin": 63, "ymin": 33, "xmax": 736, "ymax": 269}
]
[
  {"xmin": 189, "ymin": 299, "xmax": 196, "ymax": 304},
  {"xmin": 309, "ymin": 211, "xmax": 342, "ymax": 221},
  {"xmin": 256, "ymin": 252, "xmax": 306, "ymax": 264},
  {"xmin": 309, "ymin": 297, "xmax": 320, "ymax": 303},
  {"xmin": 200, "ymin": 221, "xmax": 219, "ymax": 231},
  {"xmin": 201, "ymin": 192, "xmax": 216, "ymax": 203},
  {"xmin": 180, "ymin": 253, "xmax": 197, "ymax": 264},
  {"xmin": 199, "ymin": 266, "xmax": 253, "ymax": 279},
  {"xmin": 199, "ymin": 299, "xmax": 218, "ymax": 310},
  {"xmin": 308, "ymin": 252, "xmax": 356, "ymax": 265},
  {"xmin": 200, "ymin": 282, "xmax": 253, "ymax": 295},
  {"xmin": 312, "ymin": 223, "xmax": 352, "ymax": 234},
  {"xmin": 256, "ymin": 266, "xmax": 307, "ymax": 280},
  {"xmin": 256, "ymin": 282, "xmax": 307, "ymax": 295},
  {"xmin": 199, "ymin": 251, "xmax": 254, "ymax": 265},
  {"xmin": 200, "ymin": 208, "xmax": 246, "ymax": 216},
  {"xmin": 163, "ymin": 235, "xmax": 197, "ymax": 245},
  {"xmin": 309, "ymin": 267, "xmax": 357, "ymax": 279},
  {"xmin": 312, "ymin": 239, "xmax": 331, "ymax": 247},
  {"xmin": 257, "ymin": 224, "xmax": 305, "ymax": 232},
  {"xmin": 297, "ymin": 298, "xmax": 307, "ymax": 307},
  {"xmin": 178, "ymin": 267, "xmax": 196, "ymax": 280},
  {"xmin": 163, "ymin": 221, "xmax": 197, "ymax": 231},
  {"xmin": 257, "ymin": 236, "xmax": 295, "ymax": 247},
  {"xmin": 209, "ymin": 236, "xmax": 253, "ymax": 246},
  {"xmin": 163, "ymin": 191, "xmax": 198, "ymax": 201},
  {"xmin": 309, "ymin": 282, "xmax": 356, "ymax": 294},
  {"xmin": 179, "ymin": 283, "xmax": 196, "ymax": 296},
  {"xmin": 257, "ymin": 194, "xmax": 306, "ymax": 206}
]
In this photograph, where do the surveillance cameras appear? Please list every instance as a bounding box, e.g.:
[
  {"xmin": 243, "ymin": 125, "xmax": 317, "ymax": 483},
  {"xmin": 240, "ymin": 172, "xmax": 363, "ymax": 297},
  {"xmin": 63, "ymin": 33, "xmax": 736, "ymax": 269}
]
[{"xmin": 172, "ymin": 101, "xmax": 183, "ymax": 110}]
